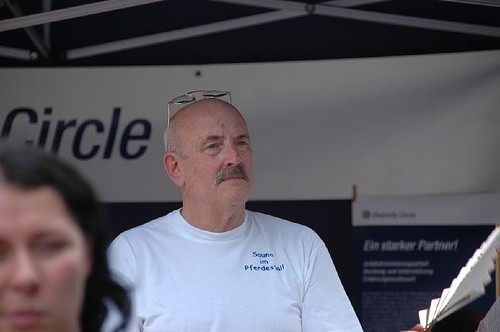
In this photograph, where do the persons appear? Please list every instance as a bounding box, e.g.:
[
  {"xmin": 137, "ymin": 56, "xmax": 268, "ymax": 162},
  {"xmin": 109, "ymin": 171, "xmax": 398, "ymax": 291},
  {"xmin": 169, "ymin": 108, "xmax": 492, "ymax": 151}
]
[
  {"xmin": 475, "ymin": 297, "xmax": 500, "ymax": 332},
  {"xmin": 101, "ymin": 89, "xmax": 431, "ymax": 332},
  {"xmin": 0, "ymin": 136, "xmax": 134, "ymax": 332}
]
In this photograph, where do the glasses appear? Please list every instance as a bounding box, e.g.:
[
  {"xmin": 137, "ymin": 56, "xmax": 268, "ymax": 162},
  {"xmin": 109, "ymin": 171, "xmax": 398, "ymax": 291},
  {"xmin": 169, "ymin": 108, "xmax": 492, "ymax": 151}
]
[{"xmin": 166, "ymin": 89, "xmax": 233, "ymax": 154}]
